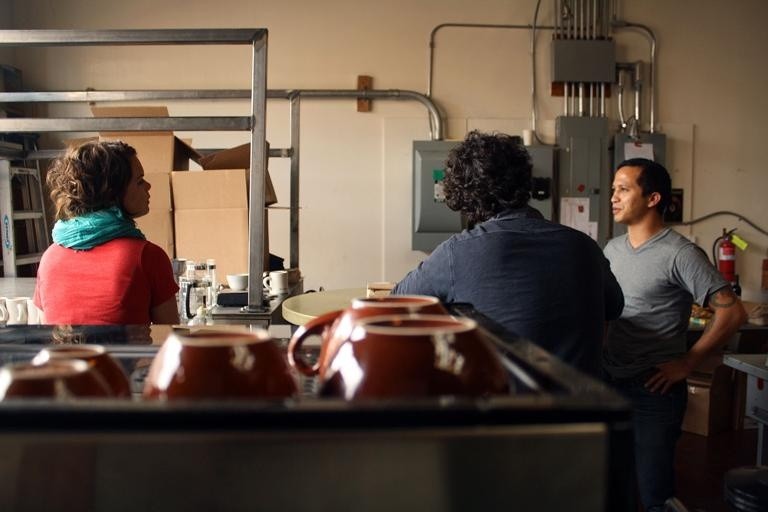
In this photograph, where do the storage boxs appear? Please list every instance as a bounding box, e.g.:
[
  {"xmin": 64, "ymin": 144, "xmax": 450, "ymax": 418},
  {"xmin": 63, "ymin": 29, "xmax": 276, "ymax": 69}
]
[{"xmin": 62, "ymin": 106, "xmax": 279, "ymax": 287}]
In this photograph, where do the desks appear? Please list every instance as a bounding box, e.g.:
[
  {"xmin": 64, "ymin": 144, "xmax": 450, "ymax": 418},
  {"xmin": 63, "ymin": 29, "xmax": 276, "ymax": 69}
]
[
  {"xmin": 672, "ymin": 314, "xmax": 768, "ymax": 465},
  {"xmin": 281, "ymin": 288, "xmax": 441, "ymax": 328}
]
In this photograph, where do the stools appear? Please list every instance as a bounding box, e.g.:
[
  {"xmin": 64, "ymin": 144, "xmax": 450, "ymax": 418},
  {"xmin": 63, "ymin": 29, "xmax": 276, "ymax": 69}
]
[{"xmin": 723, "ymin": 465, "xmax": 768, "ymax": 511}]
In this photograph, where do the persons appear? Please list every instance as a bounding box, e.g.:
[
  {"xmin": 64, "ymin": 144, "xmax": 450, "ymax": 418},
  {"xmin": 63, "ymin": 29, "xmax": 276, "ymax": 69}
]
[
  {"xmin": 601, "ymin": 156, "xmax": 749, "ymax": 511},
  {"xmin": 33, "ymin": 140, "xmax": 180, "ymax": 326},
  {"xmin": 387, "ymin": 127, "xmax": 625, "ymax": 378}
]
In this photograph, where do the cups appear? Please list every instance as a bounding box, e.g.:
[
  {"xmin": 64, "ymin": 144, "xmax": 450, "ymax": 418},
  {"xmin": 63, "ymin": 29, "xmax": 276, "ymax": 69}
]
[
  {"xmin": 4, "ymin": 363, "xmax": 111, "ymax": 400},
  {"xmin": 141, "ymin": 332, "xmax": 302, "ymax": 397},
  {"xmin": 316, "ymin": 314, "xmax": 508, "ymax": 399},
  {"xmin": 285, "ymin": 295, "xmax": 451, "ymax": 399},
  {"xmin": 225, "ymin": 273, "xmax": 250, "ymax": 292},
  {"xmin": 178, "ymin": 258, "xmax": 219, "ymax": 328},
  {"xmin": 0, "ymin": 295, "xmax": 41, "ymax": 327},
  {"xmin": 263, "ymin": 269, "xmax": 289, "ymax": 297},
  {"xmin": 30, "ymin": 347, "xmax": 135, "ymax": 400}
]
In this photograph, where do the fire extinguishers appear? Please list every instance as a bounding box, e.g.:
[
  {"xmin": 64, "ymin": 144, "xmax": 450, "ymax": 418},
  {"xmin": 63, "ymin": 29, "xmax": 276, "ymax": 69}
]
[{"xmin": 713, "ymin": 227, "xmax": 748, "ymax": 283}]
